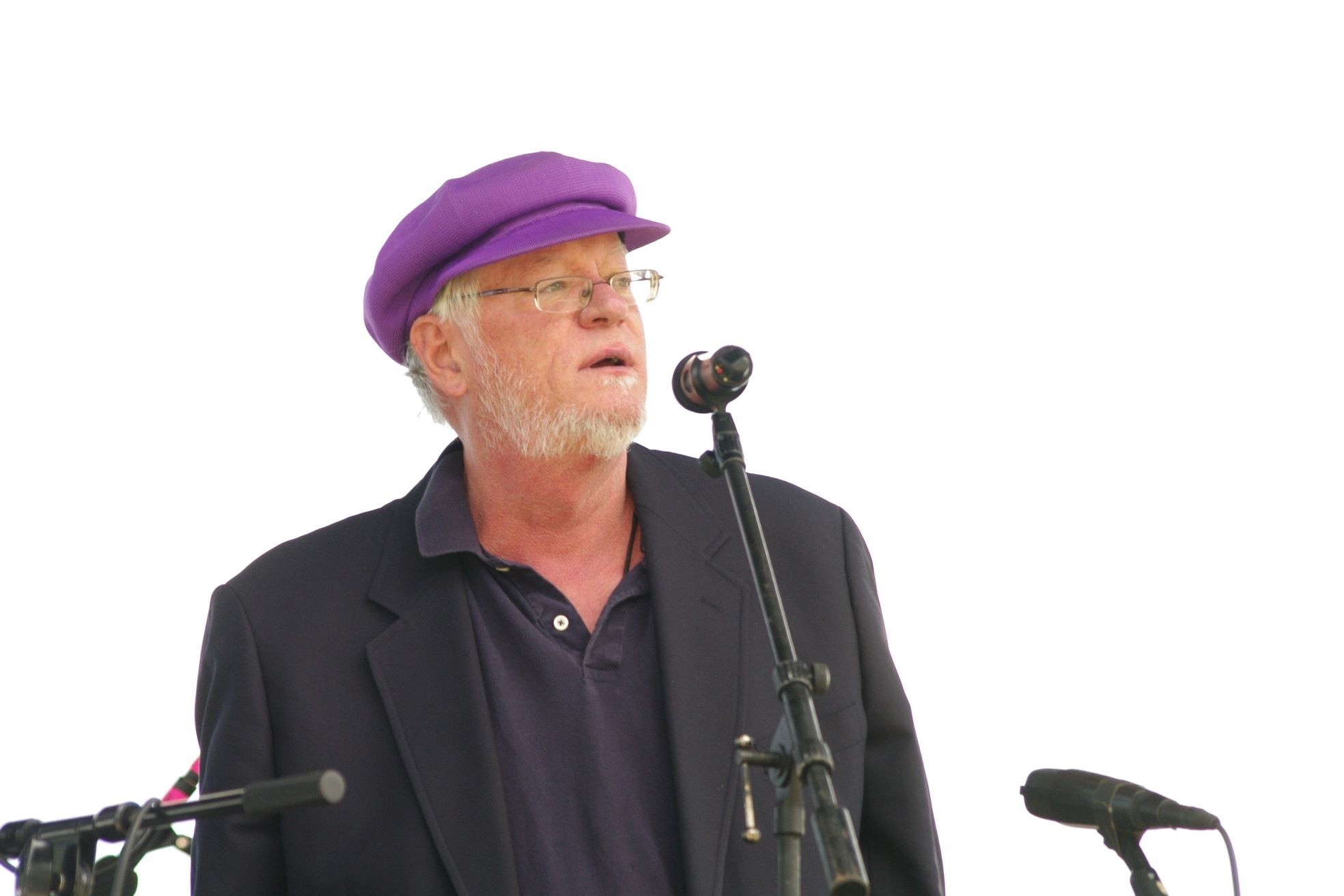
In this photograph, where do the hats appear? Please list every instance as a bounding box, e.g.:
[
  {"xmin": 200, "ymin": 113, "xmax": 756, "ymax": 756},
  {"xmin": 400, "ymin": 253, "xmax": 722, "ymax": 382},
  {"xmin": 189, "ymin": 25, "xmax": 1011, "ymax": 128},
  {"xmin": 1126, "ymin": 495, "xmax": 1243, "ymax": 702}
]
[{"xmin": 363, "ymin": 151, "xmax": 671, "ymax": 365}]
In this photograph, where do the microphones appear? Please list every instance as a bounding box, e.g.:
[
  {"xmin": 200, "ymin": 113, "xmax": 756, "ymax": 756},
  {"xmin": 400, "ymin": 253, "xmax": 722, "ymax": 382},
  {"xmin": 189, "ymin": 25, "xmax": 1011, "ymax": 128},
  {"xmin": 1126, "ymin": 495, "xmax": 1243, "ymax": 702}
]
[
  {"xmin": 91, "ymin": 762, "xmax": 200, "ymax": 895},
  {"xmin": 670, "ymin": 346, "xmax": 753, "ymax": 413},
  {"xmin": 1021, "ymin": 770, "xmax": 1221, "ymax": 834}
]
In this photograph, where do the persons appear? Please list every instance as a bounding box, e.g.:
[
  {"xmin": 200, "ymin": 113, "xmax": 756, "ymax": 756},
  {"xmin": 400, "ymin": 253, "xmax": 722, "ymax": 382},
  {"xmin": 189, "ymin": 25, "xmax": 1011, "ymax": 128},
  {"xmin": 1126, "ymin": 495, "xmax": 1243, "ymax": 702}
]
[{"xmin": 191, "ymin": 154, "xmax": 949, "ymax": 896}]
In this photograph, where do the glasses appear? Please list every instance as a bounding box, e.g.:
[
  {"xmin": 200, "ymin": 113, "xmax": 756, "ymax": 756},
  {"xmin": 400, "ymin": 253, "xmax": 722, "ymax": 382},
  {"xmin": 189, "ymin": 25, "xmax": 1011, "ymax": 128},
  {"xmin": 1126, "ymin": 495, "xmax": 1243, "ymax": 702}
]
[{"xmin": 461, "ymin": 270, "xmax": 663, "ymax": 314}]
